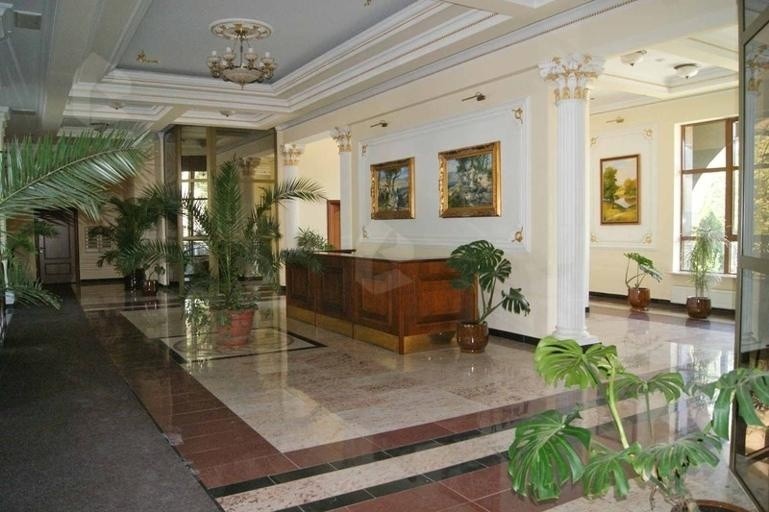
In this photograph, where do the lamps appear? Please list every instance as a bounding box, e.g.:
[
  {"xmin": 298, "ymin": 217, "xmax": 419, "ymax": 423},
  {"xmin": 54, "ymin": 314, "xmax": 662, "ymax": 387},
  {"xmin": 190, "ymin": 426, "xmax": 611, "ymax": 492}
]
[
  {"xmin": 673, "ymin": 64, "xmax": 700, "ymax": 80},
  {"xmin": 208, "ymin": 18, "xmax": 278, "ymax": 90},
  {"xmin": 621, "ymin": 50, "xmax": 647, "ymax": 67},
  {"xmin": 221, "ymin": 110, "xmax": 235, "ymax": 117},
  {"xmin": 111, "ymin": 102, "xmax": 124, "ymax": 110},
  {"xmin": 92, "ymin": 123, "xmax": 109, "ymax": 132}
]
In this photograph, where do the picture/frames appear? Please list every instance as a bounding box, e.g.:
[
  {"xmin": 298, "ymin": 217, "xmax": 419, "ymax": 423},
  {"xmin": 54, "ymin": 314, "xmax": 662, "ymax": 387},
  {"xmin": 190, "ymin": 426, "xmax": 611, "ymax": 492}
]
[
  {"xmin": 437, "ymin": 141, "xmax": 501, "ymax": 218},
  {"xmin": 370, "ymin": 156, "xmax": 415, "ymax": 220},
  {"xmin": 600, "ymin": 155, "xmax": 641, "ymax": 225}
]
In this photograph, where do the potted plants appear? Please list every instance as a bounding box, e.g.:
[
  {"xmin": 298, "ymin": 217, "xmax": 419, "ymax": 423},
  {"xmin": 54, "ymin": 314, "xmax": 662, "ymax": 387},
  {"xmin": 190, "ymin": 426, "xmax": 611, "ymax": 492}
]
[
  {"xmin": 625, "ymin": 252, "xmax": 663, "ymax": 312},
  {"xmin": 88, "ymin": 196, "xmax": 176, "ymax": 290},
  {"xmin": 687, "ymin": 213, "xmax": 732, "ymax": 320},
  {"xmin": 445, "ymin": 240, "xmax": 530, "ymax": 354},
  {"xmin": 142, "ymin": 264, "xmax": 165, "ymax": 297},
  {"xmin": 114, "ymin": 161, "xmax": 336, "ymax": 347},
  {"xmin": 508, "ymin": 336, "xmax": 769, "ymax": 512}
]
